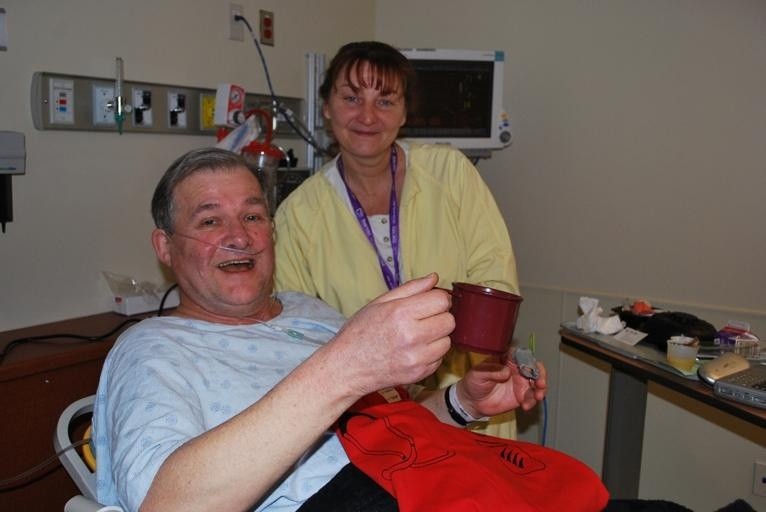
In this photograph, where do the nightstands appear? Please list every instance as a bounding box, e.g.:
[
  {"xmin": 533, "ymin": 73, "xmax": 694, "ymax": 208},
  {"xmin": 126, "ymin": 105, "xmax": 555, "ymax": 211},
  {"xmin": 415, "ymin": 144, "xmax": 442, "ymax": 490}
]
[{"xmin": 1, "ymin": 308, "xmax": 174, "ymax": 512}]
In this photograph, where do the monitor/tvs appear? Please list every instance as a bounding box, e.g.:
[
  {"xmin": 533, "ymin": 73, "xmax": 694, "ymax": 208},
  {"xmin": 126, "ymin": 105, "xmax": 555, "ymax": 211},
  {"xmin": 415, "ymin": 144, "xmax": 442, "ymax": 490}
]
[{"xmin": 395, "ymin": 46, "xmax": 513, "ymax": 151}]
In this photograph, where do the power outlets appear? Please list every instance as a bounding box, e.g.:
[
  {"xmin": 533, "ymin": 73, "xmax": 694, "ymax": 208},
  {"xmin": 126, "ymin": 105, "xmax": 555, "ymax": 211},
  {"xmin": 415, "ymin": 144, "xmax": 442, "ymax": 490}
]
[{"xmin": 227, "ymin": 5, "xmax": 245, "ymax": 43}]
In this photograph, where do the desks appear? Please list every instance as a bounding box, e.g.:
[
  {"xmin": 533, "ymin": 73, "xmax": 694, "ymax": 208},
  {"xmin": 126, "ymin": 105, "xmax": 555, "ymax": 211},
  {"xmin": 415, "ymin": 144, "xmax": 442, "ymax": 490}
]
[{"xmin": 558, "ymin": 320, "xmax": 766, "ymax": 500}]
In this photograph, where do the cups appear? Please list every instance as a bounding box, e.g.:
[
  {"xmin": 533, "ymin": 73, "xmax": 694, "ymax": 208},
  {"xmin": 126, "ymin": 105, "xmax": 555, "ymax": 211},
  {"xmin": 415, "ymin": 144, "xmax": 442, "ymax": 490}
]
[
  {"xmin": 432, "ymin": 281, "xmax": 524, "ymax": 357},
  {"xmin": 668, "ymin": 339, "xmax": 699, "ymax": 373}
]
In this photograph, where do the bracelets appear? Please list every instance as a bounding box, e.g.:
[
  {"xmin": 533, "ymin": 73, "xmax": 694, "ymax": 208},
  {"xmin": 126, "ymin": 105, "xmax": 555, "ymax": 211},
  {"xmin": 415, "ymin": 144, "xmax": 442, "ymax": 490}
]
[{"xmin": 444, "ymin": 383, "xmax": 469, "ymax": 426}]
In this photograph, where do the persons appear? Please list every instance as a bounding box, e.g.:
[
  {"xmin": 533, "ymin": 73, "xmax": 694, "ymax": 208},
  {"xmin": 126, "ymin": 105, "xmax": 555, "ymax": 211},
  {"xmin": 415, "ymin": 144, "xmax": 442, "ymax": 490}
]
[
  {"xmin": 91, "ymin": 147, "xmax": 757, "ymax": 512},
  {"xmin": 272, "ymin": 42, "xmax": 520, "ymax": 441}
]
